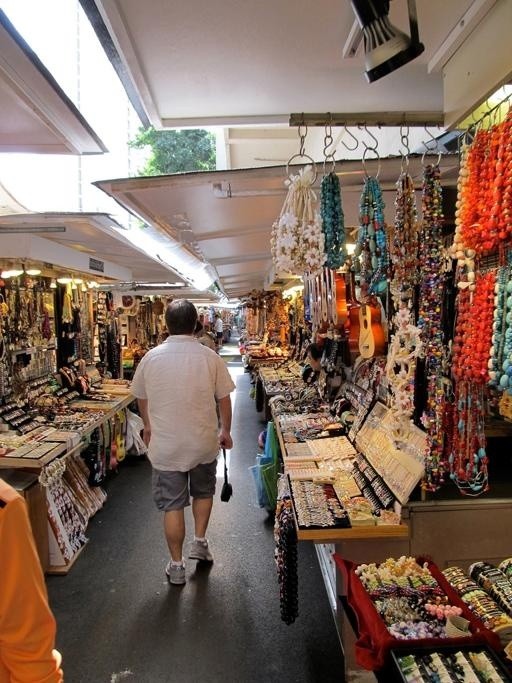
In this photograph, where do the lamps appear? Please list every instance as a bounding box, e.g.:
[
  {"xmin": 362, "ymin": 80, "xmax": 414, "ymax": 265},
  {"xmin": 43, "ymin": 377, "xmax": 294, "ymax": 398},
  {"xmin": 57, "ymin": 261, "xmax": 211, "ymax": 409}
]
[{"xmin": 348, "ymin": 1, "xmax": 425, "ymax": 85}]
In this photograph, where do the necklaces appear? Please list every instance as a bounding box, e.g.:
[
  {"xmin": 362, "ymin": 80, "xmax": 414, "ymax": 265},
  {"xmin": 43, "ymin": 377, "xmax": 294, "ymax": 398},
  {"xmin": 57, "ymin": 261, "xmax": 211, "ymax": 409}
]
[
  {"xmin": 1, "ymin": 283, "xmax": 57, "ymax": 343},
  {"xmin": 384, "ymin": 113, "xmax": 512, "ymax": 497},
  {"xmin": 352, "ymin": 177, "xmax": 390, "ymax": 295},
  {"xmin": 62, "ymin": 294, "xmax": 73, "ymax": 323},
  {"xmin": 318, "ymin": 171, "xmax": 347, "ymax": 270}
]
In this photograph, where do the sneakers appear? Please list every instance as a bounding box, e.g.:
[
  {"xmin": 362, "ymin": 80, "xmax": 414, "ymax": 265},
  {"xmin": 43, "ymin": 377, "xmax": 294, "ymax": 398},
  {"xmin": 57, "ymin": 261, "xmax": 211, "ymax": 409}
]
[
  {"xmin": 165, "ymin": 558, "xmax": 187, "ymax": 585},
  {"xmin": 187, "ymin": 538, "xmax": 213, "ymax": 562}
]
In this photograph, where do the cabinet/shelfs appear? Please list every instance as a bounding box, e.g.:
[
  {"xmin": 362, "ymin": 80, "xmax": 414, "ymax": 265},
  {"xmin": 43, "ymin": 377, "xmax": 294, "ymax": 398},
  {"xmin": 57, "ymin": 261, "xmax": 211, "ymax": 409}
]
[
  {"xmin": 112, "ymin": 282, "xmax": 206, "ymax": 382},
  {"xmin": 241, "ymin": 300, "xmax": 297, "ymax": 370},
  {"xmin": 254, "ymin": 266, "xmax": 358, "ymax": 422},
  {"xmin": 0, "ymin": 255, "xmax": 136, "ymax": 575},
  {"xmin": 271, "ymin": 228, "xmax": 511, "ymax": 612},
  {"xmin": 339, "ymin": 551, "xmax": 511, "ymax": 683}
]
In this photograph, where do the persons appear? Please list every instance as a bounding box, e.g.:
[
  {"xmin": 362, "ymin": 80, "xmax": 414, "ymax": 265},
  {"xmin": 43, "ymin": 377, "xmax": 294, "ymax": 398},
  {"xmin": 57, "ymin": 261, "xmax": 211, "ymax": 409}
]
[
  {"xmin": 132, "ymin": 300, "xmax": 236, "ymax": 586},
  {"xmin": 306, "ymin": 342, "xmax": 324, "ymax": 371},
  {"xmin": 214, "ymin": 315, "xmax": 223, "ymax": 346},
  {"xmin": 0, "ymin": 482, "xmax": 67, "ymax": 681}
]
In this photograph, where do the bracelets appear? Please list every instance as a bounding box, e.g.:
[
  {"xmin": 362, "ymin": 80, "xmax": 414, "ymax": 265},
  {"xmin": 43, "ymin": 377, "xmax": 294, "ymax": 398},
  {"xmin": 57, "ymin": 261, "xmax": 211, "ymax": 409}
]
[{"xmin": 358, "ymin": 555, "xmax": 512, "ymax": 639}]
